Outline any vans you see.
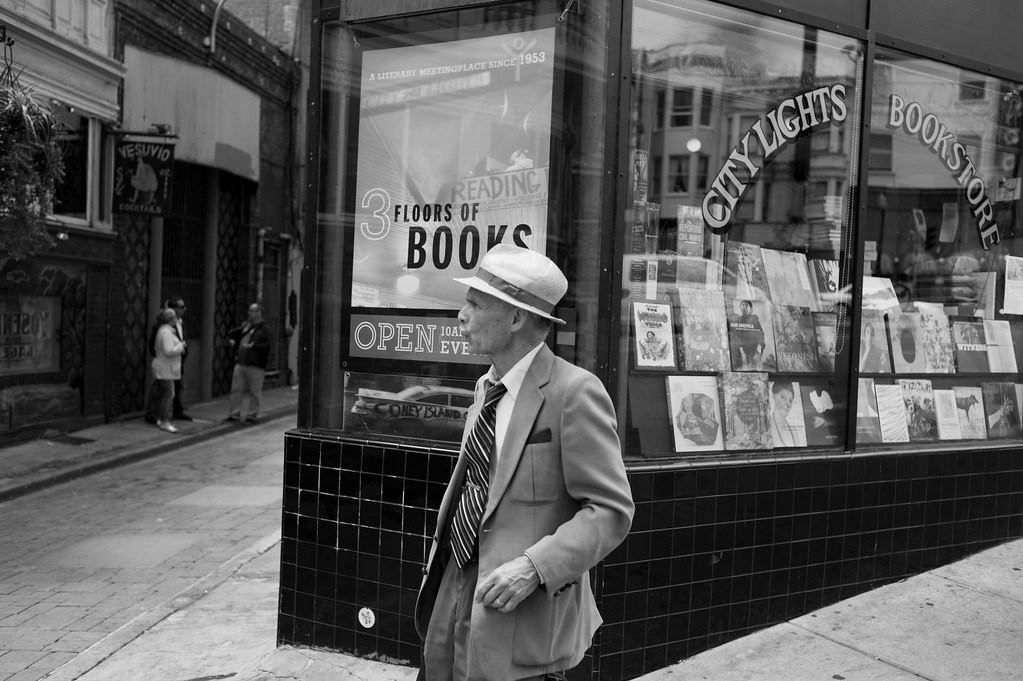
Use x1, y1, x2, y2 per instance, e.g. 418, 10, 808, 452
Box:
622, 253, 774, 335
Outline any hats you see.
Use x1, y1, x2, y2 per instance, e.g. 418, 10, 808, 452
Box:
454, 243, 569, 325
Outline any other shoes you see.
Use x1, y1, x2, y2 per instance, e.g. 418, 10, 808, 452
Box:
149, 408, 254, 432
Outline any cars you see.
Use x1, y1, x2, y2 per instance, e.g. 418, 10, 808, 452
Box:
351, 383, 474, 440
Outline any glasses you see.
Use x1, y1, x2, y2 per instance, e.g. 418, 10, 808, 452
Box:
176, 305, 187, 309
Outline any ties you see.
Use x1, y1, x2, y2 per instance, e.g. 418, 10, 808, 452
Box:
449, 384, 508, 567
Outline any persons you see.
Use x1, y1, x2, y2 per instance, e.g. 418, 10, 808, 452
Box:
858, 322, 885, 373
152, 308, 189, 435
222, 302, 273, 426
413, 243, 637, 681
146, 295, 194, 425
